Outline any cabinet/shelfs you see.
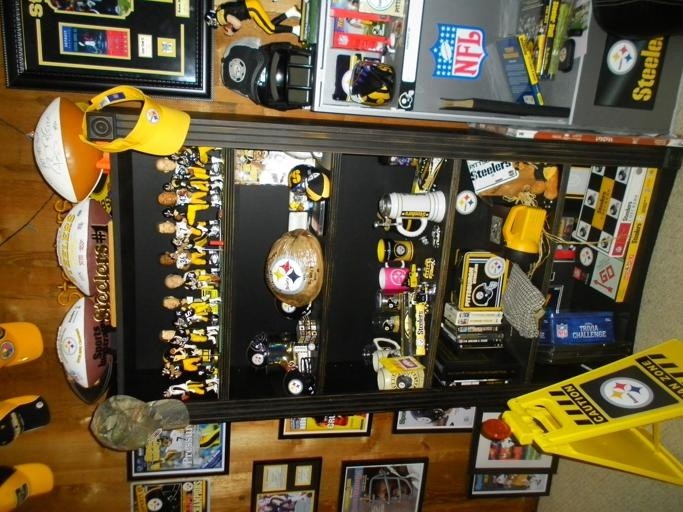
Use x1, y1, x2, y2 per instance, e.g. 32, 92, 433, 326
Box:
90, 113, 683, 422
310, 26, 681, 140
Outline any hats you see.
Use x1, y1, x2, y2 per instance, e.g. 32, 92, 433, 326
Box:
0, 321, 43, 369
78, 84, 191, 156
219, 37, 269, 103
0, 463, 54, 512
88, 395, 191, 453
0, 395, 51, 448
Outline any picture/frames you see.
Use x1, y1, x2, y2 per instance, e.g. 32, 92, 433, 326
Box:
1, 4, 211, 98
127, 407, 559, 511
